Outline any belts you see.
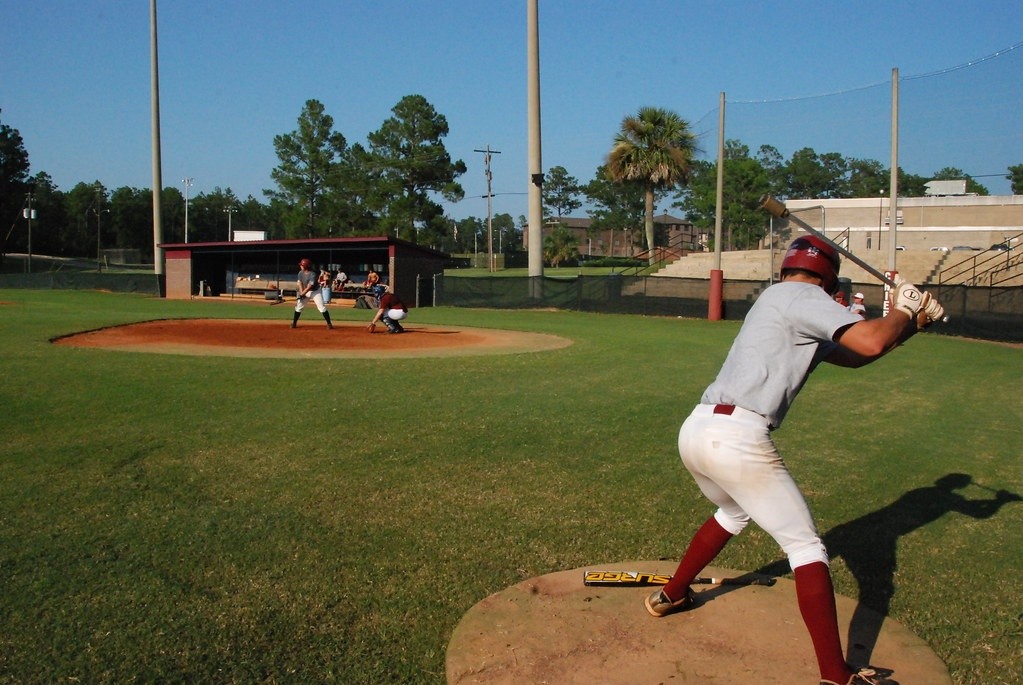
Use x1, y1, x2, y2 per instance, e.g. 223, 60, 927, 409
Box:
714, 404, 735, 415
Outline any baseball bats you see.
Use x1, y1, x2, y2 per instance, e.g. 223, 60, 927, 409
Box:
758, 190, 951, 322
583, 570, 775, 587
269, 298, 300, 306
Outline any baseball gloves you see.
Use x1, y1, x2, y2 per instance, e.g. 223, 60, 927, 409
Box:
367, 323, 376, 333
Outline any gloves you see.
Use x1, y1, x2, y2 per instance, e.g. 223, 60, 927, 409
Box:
917, 290, 943, 328
891, 280, 922, 320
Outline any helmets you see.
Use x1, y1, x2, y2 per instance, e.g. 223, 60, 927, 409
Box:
779, 234, 841, 296
371, 286, 385, 298
299, 259, 310, 268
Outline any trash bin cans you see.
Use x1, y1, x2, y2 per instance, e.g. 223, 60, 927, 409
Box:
200, 280, 207, 296
323, 288, 331, 304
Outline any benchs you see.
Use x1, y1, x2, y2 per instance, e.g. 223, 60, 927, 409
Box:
234, 280, 382, 298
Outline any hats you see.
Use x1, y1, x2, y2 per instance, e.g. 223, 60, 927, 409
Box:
836, 291, 844, 298
854, 293, 864, 299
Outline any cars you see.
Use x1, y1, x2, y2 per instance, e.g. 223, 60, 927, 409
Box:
450, 260, 469, 269
897, 244, 1013, 251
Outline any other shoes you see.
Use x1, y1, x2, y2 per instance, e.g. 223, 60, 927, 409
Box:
328, 323, 334, 329
292, 324, 297, 329
386, 329, 404, 334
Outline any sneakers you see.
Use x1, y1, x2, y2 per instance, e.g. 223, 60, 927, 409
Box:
820, 668, 889, 685
644, 585, 698, 617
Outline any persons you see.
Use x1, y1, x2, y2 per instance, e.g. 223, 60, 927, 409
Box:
367, 285, 409, 333
646, 235, 945, 685
835, 291, 848, 308
363, 269, 379, 289
333, 268, 347, 291
318, 265, 331, 304
290, 259, 333, 330
850, 293, 866, 318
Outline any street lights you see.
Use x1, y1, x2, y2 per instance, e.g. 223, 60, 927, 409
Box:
394, 228, 398, 237
97, 210, 110, 272
222, 209, 238, 242
494, 229, 507, 253
182, 178, 195, 243
878, 190, 884, 250
